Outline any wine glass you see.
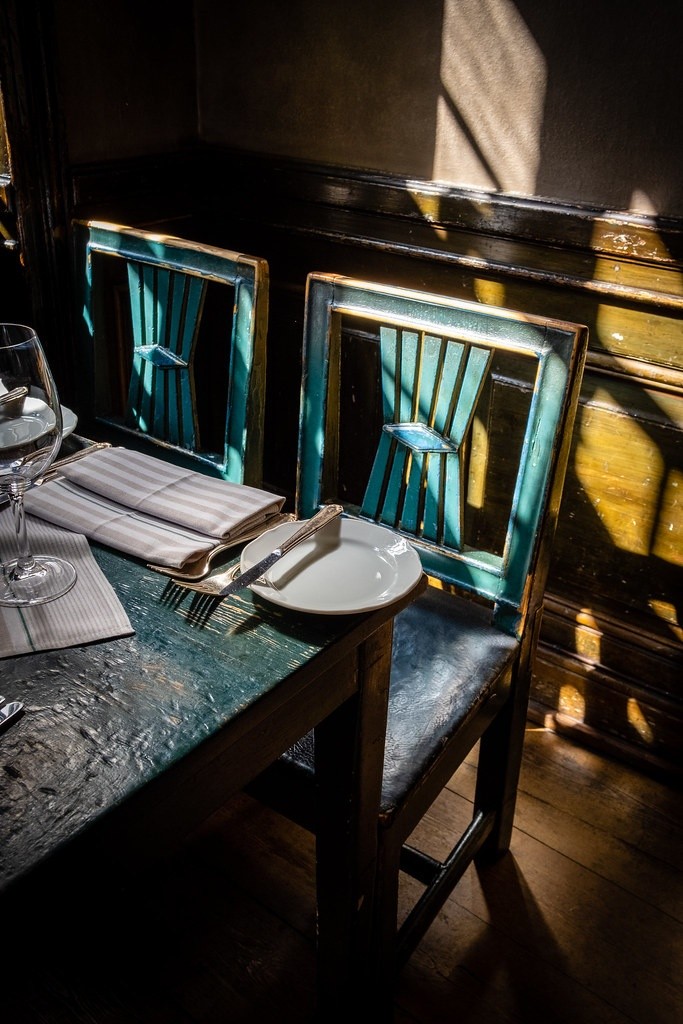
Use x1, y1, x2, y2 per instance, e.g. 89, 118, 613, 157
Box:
0, 324, 77, 609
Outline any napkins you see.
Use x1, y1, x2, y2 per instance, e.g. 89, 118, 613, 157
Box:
1, 445, 294, 659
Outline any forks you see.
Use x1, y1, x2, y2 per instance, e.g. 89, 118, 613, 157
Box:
171, 562, 238, 596
147, 513, 299, 581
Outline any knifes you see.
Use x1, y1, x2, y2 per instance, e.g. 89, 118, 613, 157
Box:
216, 504, 344, 595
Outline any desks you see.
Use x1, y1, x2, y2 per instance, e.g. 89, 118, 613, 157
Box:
0, 435, 431, 1024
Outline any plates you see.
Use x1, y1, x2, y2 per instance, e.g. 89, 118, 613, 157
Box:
0, 396, 78, 449
239, 518, 423, 615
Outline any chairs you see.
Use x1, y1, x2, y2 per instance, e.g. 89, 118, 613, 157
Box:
228, 274, 593, 998
60, 216, 269, 499
